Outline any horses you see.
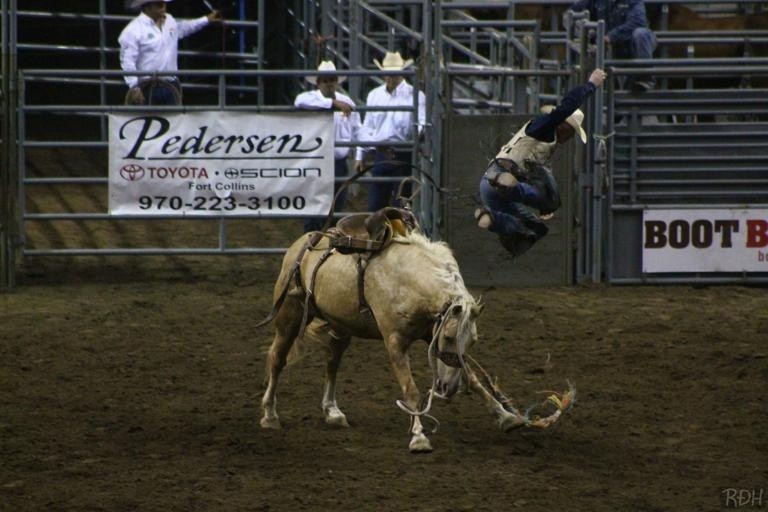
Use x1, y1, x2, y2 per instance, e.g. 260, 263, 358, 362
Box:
258, 228, 527, 453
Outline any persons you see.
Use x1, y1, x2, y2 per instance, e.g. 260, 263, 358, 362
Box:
117, 0, 222, 106
570, 0, 659, 90
361, 51, 426, 220
474, 68, 607, 259
294, 60, 362, 234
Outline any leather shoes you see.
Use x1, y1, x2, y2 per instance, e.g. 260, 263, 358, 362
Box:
474, 208, 494, 229
486, 171, 519, 191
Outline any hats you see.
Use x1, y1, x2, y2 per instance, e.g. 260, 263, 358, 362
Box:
130, 0, 170, 9
373, 52, 414, 70
540, 105, 587, 144
304, 61, 348, 86
